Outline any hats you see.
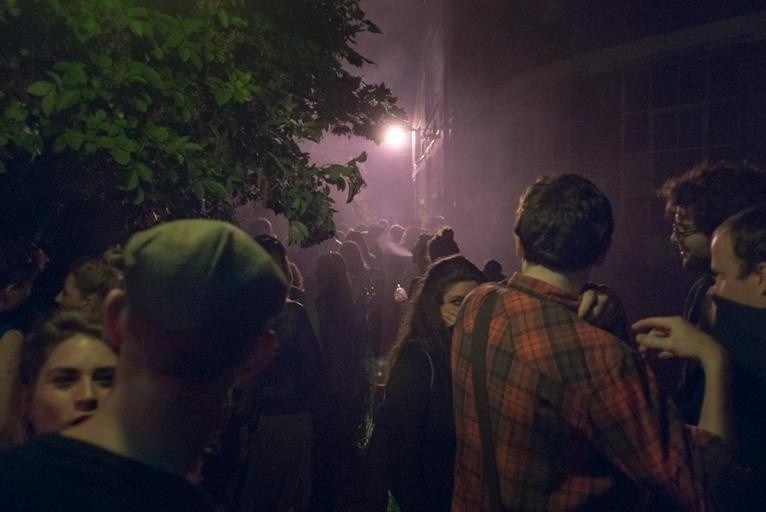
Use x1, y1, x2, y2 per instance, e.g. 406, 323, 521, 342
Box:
125, 218, 288, 340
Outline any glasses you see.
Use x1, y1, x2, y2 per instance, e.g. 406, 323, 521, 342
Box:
673, 223, 701, 235
